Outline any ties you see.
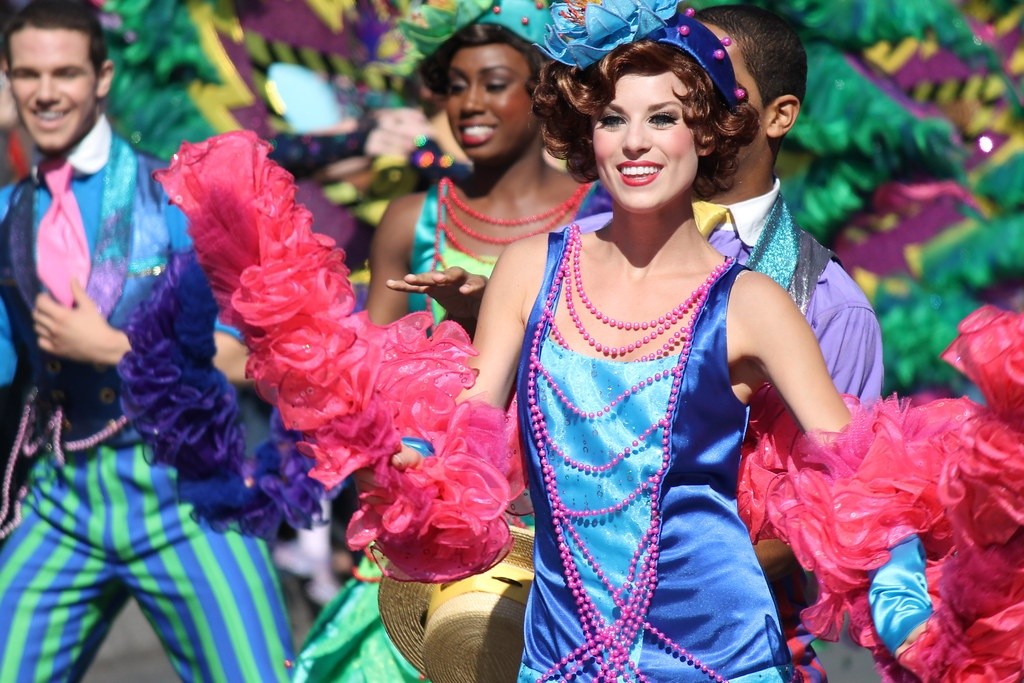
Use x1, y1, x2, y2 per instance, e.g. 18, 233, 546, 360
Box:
37, 160, 90, 310
691, 197, 733, 242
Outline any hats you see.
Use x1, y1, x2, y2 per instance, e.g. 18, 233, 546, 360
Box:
378, 526, 536, 683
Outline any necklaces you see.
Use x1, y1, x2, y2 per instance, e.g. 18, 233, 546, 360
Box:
426, 174, 590, 337
529, 224, 734, 683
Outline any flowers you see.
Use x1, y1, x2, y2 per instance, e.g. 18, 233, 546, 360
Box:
370, 1, 496, 78
530, 0, 677, 69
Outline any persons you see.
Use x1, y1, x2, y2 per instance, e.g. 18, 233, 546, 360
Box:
296, 23, 595, 683
569, 4, 884, 580
0, 0, 298, 683
393, 15, 937, 683
